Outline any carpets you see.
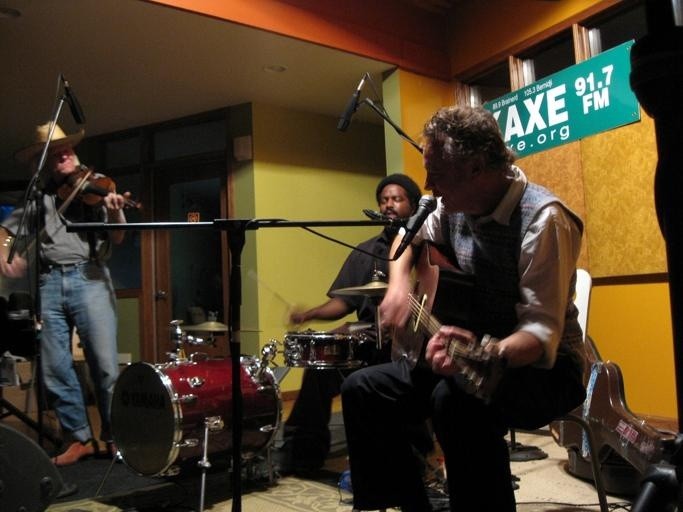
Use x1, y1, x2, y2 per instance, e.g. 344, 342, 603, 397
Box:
45, 412, 633, 511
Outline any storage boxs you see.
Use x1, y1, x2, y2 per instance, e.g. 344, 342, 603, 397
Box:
0, 359, 43, 413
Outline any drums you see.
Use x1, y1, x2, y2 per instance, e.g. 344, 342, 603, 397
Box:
281, 329, 368, 370
109, 352, 281, 480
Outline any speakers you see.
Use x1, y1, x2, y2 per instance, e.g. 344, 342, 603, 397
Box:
0, 423, 64, 512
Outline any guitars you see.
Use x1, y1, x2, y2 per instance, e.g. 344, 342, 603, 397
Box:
391, 243, 511, 405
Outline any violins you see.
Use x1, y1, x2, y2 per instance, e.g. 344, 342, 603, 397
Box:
57, 171, 143, 213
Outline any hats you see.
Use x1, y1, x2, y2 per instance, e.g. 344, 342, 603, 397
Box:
376, 174, 421, 215
18, 120, 84, 158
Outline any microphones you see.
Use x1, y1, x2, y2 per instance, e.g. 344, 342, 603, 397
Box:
393, 194, 437, 260
61, 73, 85, 124
336, 73, 367, 133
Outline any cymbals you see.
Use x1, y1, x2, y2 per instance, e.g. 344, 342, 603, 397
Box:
161, 321, 265, 333
328, 282, 388, 297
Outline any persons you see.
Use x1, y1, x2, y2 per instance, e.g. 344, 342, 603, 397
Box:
628, 0, 683, 430
0, 120, 133, 465
280, 173, 438, 475
341, 103, 586, 512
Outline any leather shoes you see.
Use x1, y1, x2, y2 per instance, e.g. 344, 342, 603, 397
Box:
108, 443, 118, 458
51, 443, 100, 466
